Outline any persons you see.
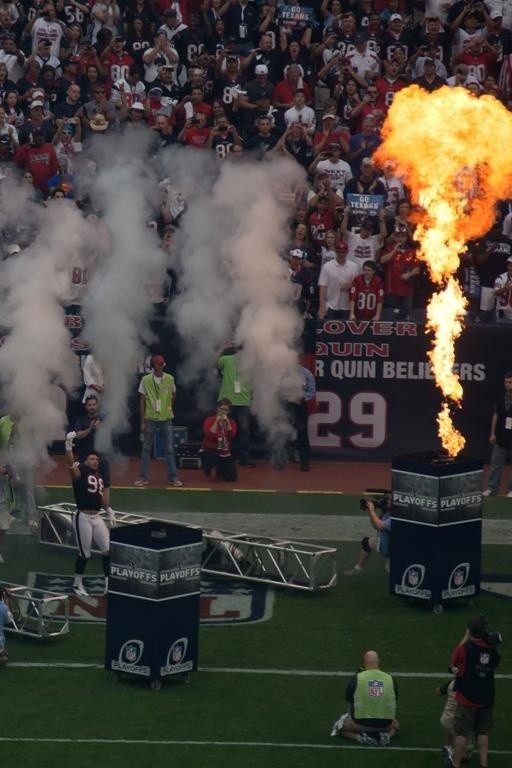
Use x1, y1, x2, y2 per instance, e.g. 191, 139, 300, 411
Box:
316, 240, 358, 319
343, 492, 393, 576
466, 82, 481, 97
272, 65, 316, 109
49, 188, 66, 203
0, 398, 60, 528
474, 224, 512, 324
77, 65, 105, 98
0, 106, 19, 156
292, 202, 307, 223
124, 14, 155, 65
340, 206, 387, 274
61, 21, 87, 58
177, 112, 210, 150
64, 431, 116, 596
380, 0, 405, 19
244, 64, 275, 102
15, 168, 44, 204
123, 0, 154, 33
283, 88, 316, 133
348, 261, 384, 321
3, 91, 23, 126
161, 225, 179, 246
176, 89, 211, 120
321, 230, 339, 263
243, 33, 278, 80
0, 62, 16, 91
350, 117, 382, 160
0, 39, 24, 66
314, 114, 350, 155
247, 117, 279, 152
119, 102, 146, 128
210, 340, 256, 469
28, 39, 61, 79
338, 650, 400, 748
413, 15, 453, 52
111, 65, 145, 112
435, 677, 475, 768
134, 355, 184, 487
199, 398, 238, 482
351, 84, 387, 135
249, 4, 280, 51
51, 124, 83, 175
387, 198, 417, 226
379, 225, 420, 321
52, 55, 82, 117
98, 35, 134, 76
206, 114, 242, 161
25, 93, 50, 125
318, 55, 367, 100
52, 84, 83, 129
72, 395, 111, 509
320, 1, 348, 26
282, 0, 307, 20
204, 1, 226, 33
278, 21, 313, 79
14, 130, 59, 195
492, 255, 512, 324
314, 26, 345, 62
481, 372, 512, 498
151, 113, 176, 147
409, 43, 447, 81
75, 85, 120, 139
317, 98, 347, 138
145, 86, 176, 126
331, 12, 360, 41
391, 46, 408, 59
451, 2, 494, 63
19, 101, 52, 143
47, 158, 79, 203
354, 1, 379, 29
213, 48, 243, 99
413, 59, 448, 90
343, 157, 387, 234
338, 79, 368, 120
42, 66, 57, 92
149, 65, 179, 99
274, 122, 314, 160
308, 143, 355, 200
0, 2, 29, 47
377, 160, 405, 199
451, 617, 501, 768
455, 36, 497, 88
209, 99, 238, 127
180, 0, 211, 69
142, 29, 179, 87
31, 5, 67, 60
157, 8, 190, 63
86, 3, 119, 45
222, 1, 256, 47
373, 59, 406, 113
485, 11, 512, 54
276, 353, 315, 472
15, 60, 41, 94
445, 64, 469, 88
179, 69, 212, 99
484, 75, 496, 89
360, 11, 384, 42
68, 39, 100, 79
282, 249, 314, 319
384, 13, 414, 55
81, 353, 105, 415
281, 223, 314, 267
343, 32, 380, 81
309, 180, 337, 231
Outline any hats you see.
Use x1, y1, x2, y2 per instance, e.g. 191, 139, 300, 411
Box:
489, 9, 502, 19
88, 113, 109, 131
162, 8, 177, 17
335, 242, 347, 252
322, 25, 334, 35
31, 100, 43, 109
427, 40, 439, 48
150, 355, 164, 365
290, 249, 303, 258
383, 160, 395, 167
129, 101, 145, 110
425, 56, 434, 64
322, 112, 335, 120
354, 31, 368, 43
254, 64, 268, 74
390, 13, 401, 22
466, 618, 487, 638
8, 243, 20, 255
394, 225, 407, 236
32, 91, 44, 99
63, 124, 72, 130
361, 219, 374, 231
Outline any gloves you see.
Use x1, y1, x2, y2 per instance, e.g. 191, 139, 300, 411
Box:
65, 430, 76, 451
107, 507, 116, 526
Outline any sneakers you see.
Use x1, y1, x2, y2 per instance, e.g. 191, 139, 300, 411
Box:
442, 745, 454, 768
482, 488, 496, 496
168, 480, 183, 487
343, 566, 364, 577
506, 490, 512, 498
72, 584, 89, 597
357, 731, 378, 747
134, 480, 149, 486
378, 732, 390, 746
28, 520, 37, 527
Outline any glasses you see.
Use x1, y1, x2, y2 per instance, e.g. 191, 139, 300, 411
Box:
95, 90, 105, 94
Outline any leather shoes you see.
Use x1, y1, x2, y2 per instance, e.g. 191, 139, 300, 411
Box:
240, 461, 256, 467
301, 464, 309, 471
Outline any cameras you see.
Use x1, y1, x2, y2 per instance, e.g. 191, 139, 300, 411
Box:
479, 609, 502, 646
360, 489, 392, 512
230, 340, 241, 348
